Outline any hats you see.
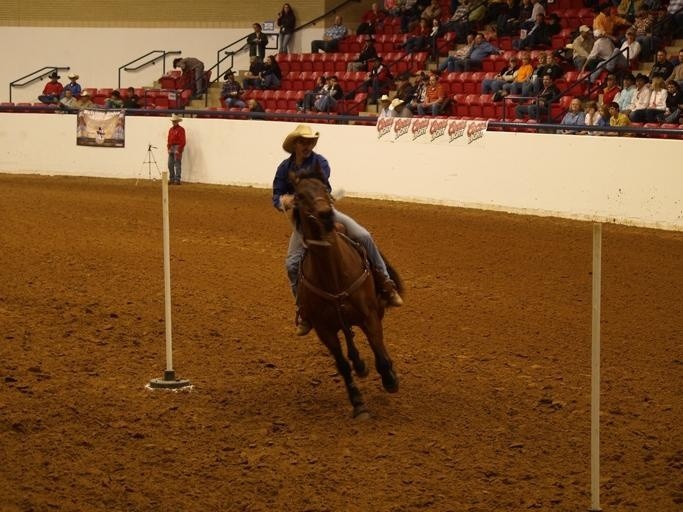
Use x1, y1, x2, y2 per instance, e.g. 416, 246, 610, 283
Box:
67, 73, 79, 79
172, 57, 182, 68
579, 24, 590, 33
224, 70, 237, 80
281, 124, 320, 152
169, 113, 183, 122
48, 73, 60, 79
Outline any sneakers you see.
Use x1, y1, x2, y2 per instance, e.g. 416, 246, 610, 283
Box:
167, 180, 181, 185
381, 281, 404, 307
295, 320, 312, 337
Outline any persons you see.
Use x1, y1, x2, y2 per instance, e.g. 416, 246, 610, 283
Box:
123, 87, 140, 114
356, 1, 440, 52
296, 75, 343, 112
174, 57, 204, 100
38, 72, 94, 114
272, 125, 404, 336
278, 3, 295, 54
377, 70, 446, 117
104, 91, 123, 112
167, 115, 185, 185
221, 23, 281, 117
556, 1, 683, 135
368, 58, 393, 97
347, 41, 376, 73
430, 1, 562, 121
311, 15, 346, 53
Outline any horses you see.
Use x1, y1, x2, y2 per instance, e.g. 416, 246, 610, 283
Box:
287, 155, 404, 423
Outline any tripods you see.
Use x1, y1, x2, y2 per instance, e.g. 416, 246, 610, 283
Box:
135, 144, 162, 185
